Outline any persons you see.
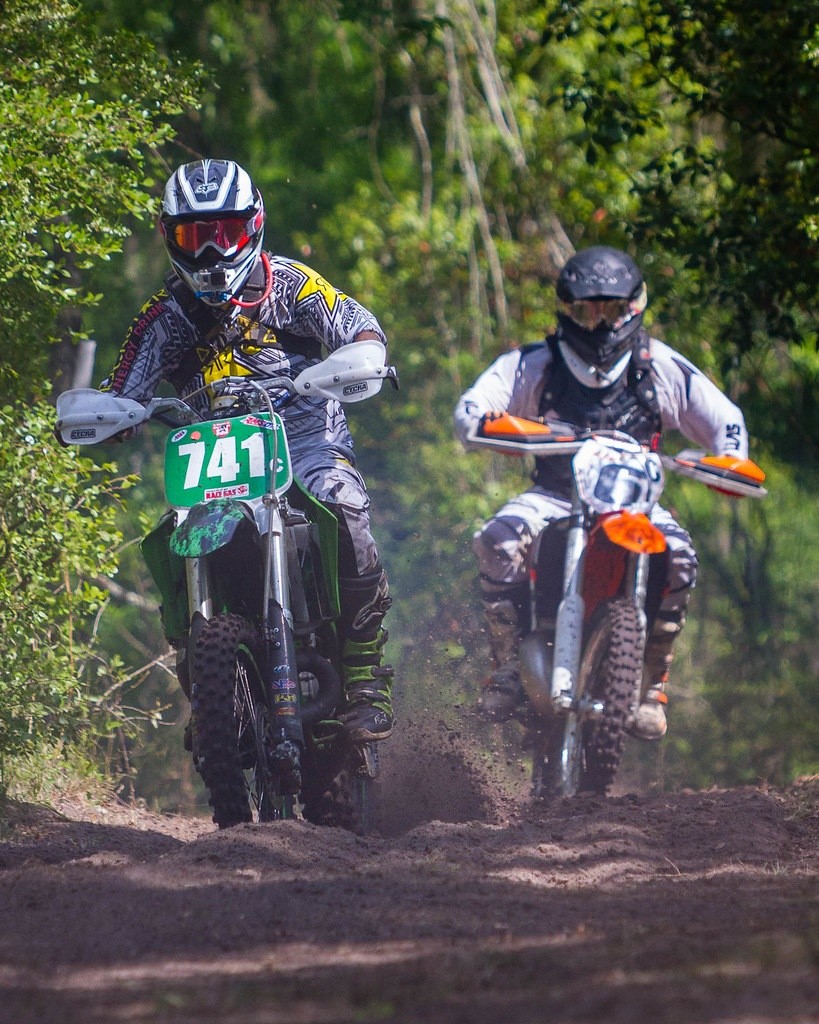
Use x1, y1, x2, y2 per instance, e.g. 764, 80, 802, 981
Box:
455, 244, 750, 741
54, 155, 396, 748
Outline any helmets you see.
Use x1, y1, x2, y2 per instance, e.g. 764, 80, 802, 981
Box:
554, 246, 648, 406
158, 158, 264, 307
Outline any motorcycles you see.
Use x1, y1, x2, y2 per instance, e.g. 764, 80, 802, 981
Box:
466, 411, 769, 800
53, 341, 403, 827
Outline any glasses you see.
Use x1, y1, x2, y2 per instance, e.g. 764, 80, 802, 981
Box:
568, 298, 631, 331
161, 207, 253, 259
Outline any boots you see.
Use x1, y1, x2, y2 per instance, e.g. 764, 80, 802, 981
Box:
176, 639, 193, 751
479, 574, 533, 718
336, 567, 395, 743
632, 610, 687, 743
